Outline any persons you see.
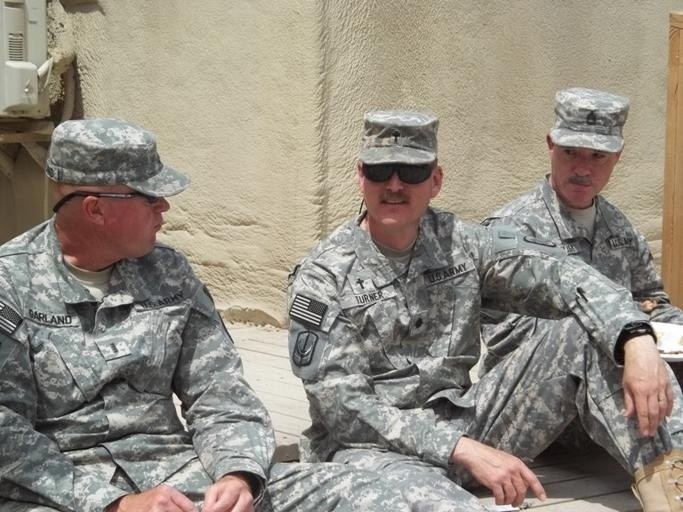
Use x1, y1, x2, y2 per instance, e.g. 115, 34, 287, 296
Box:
284, 110, 681, 511
474, 86, 683, 474
0, 116, 411, 511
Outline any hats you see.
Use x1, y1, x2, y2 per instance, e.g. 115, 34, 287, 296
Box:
44, 117, 191, 198
549, 86, 630, 153
360, 110, 439, 166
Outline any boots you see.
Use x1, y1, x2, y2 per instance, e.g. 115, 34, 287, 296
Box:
630, 448, 683, 512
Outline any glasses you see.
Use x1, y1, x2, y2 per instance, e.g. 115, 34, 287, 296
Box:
52, 190, 159, 213
362, 163, 432, 184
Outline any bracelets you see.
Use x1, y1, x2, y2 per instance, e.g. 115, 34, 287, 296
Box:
615, 321, 657, 365
